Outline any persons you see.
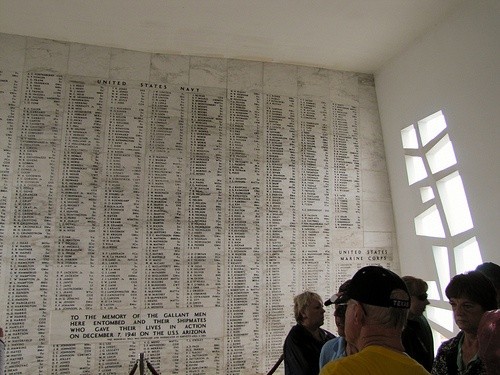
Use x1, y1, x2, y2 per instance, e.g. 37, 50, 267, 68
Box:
282, 290, 337, 375
433, 270, 500, 375
318, 265, 435, 375
474, 261, 500, 310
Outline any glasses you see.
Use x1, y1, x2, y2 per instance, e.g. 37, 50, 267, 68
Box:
414, 294, 427, 301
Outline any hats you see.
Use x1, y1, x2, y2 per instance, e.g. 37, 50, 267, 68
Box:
324, 266, 411, 309
